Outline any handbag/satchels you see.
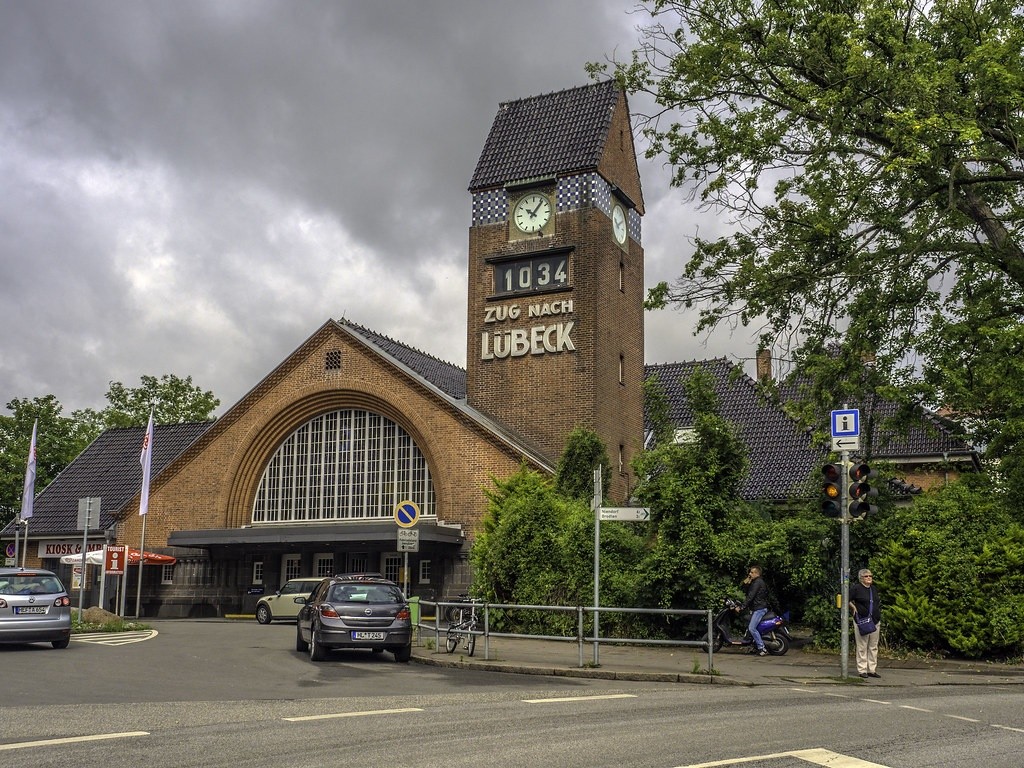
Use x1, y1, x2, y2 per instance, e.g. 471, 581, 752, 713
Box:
857, 616, 877, 636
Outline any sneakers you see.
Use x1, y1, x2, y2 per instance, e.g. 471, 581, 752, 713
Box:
754, 648, 769, 656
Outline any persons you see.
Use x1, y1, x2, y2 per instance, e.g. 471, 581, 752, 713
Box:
736, 565, 772, 657
848, 569, 883, 679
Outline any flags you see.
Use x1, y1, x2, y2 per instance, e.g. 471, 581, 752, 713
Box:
20, 417, 37, 523
138, 407, 153, 516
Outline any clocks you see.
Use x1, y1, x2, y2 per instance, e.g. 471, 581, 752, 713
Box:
609, 193, 631, 255
508, 184, 556, 242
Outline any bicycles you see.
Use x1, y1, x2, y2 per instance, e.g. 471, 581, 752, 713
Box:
446, 598, 482, 656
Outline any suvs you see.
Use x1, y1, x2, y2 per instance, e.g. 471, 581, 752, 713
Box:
0, 567, 72, 649
294, 574, 412, 664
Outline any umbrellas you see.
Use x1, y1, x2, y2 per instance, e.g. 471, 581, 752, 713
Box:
60, 545, 177, 616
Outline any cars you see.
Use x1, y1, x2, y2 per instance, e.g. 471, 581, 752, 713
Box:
254, 577, 330, 624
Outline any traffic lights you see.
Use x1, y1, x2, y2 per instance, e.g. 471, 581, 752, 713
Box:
845, 464, 879, 521
821, 464, 842, 518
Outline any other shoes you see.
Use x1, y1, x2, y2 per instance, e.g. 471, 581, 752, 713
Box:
860, 673, 881, 678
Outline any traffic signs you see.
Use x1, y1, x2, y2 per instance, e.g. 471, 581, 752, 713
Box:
831, 409, 860, 452
5, 558, 15, 566
599, 506, 651, 522
397, 527, 419, 552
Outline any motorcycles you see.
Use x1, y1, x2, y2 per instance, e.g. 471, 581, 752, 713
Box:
702, 599, 792, 656
